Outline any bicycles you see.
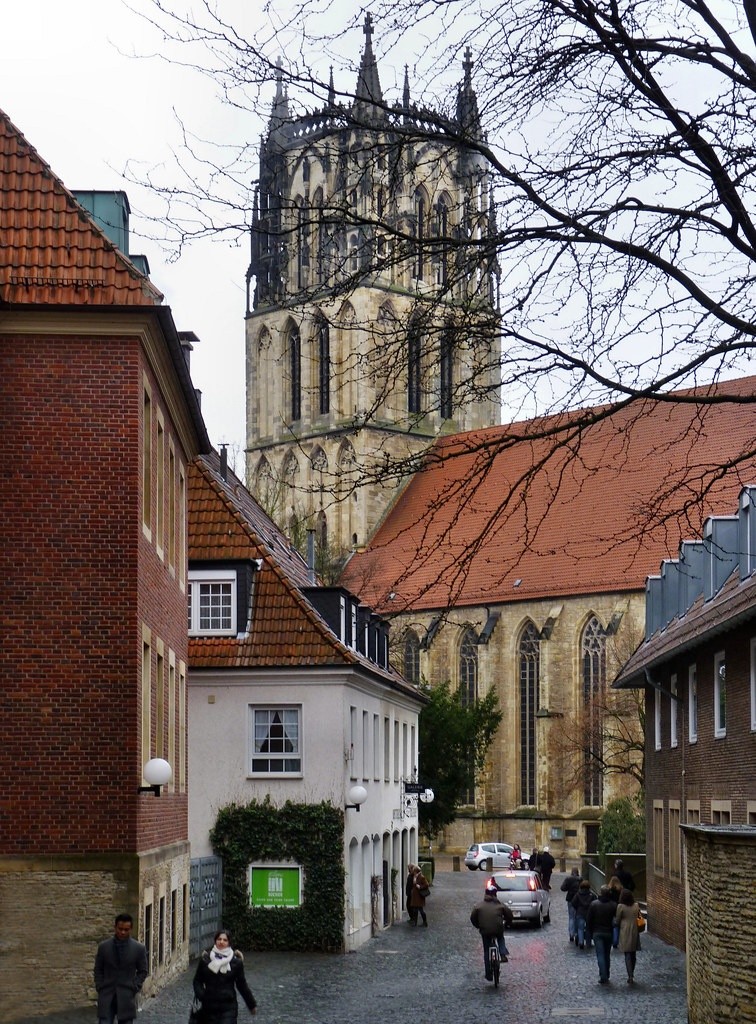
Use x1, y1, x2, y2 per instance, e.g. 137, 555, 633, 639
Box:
487, 936, 504, 989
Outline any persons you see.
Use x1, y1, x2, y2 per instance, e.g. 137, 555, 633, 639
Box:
193, 930, 258, 1024
508, 844, 555, 892
470, 885, 513, 981
406, 863, 430, 927
94, 913, 149, 1024
560, 865, 644, 986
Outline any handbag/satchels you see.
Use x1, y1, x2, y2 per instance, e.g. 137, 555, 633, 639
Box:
419, 887, 430, 897
533, 866, 541, 873
544, 847, 549, 852
188, 996, 201, 1024
637, 911, 645, 932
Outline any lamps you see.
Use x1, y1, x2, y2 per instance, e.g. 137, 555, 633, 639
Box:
344, 786, 367, 812
138, 759, 172, 797
412, 790, 434, 807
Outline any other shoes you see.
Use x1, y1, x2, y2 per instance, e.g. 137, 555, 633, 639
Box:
407, 919, 428, 927
485, 975, 493, 981
598, 979, 609, 984
502, 957, 508, 962
570, 935, 585, 950
627, 979, 633, 983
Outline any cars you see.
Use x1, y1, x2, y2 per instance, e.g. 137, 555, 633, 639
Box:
484, 870, 552, 929
465, 842, 532, 872
